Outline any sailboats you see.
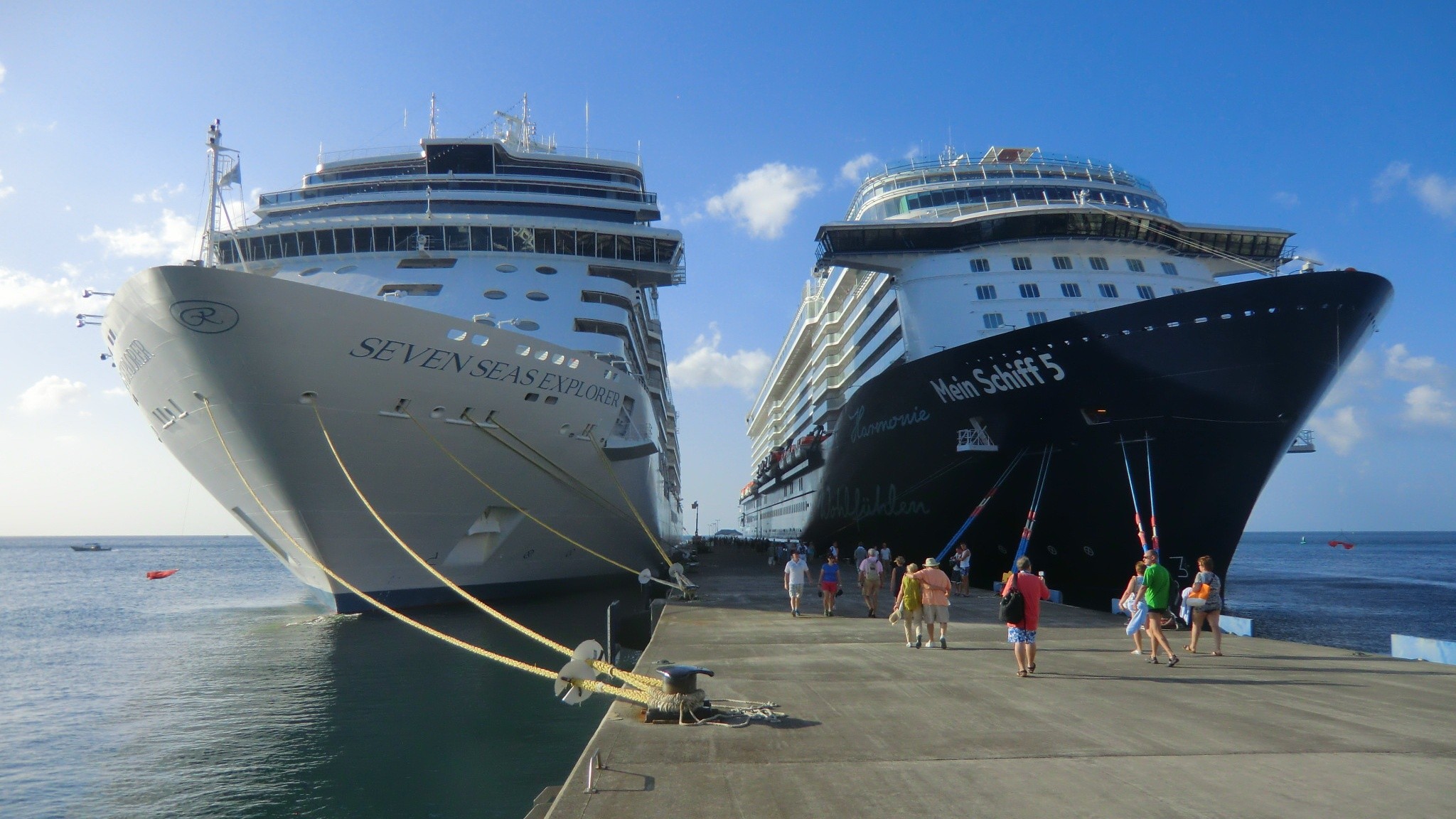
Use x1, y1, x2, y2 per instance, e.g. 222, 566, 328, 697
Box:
1300, 535, 1306, 544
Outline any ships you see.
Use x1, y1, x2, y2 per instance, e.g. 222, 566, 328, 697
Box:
78, 93, 691, 615
739, 142, 1393, 627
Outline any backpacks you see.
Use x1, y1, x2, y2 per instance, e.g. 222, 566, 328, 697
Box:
866, 558, 879, 581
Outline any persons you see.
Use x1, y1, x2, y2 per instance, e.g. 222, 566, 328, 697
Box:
1183, 555, 1223, 657
702, 536, 971, 649
1119, 550, 1180, 667
999, 557, 1050, 677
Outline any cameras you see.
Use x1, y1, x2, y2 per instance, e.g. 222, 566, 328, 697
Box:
1039, 571, 1044, 576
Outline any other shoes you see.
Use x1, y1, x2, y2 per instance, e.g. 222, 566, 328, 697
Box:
1144, 656, 1158, 664
824, 610, 833, 617
1130, 649, 1144, 654
791, 608, 800, 616
916, 633, 922, 648
1017, 670, 1027, 677
940, 636, 946, 649
868, 608, 876, 618
906, 642, 912, 647
1027, 662, 1035, 673
1212, 650, 1224, 656
1165, 654, 1179, 667
925, 642, 935, 647
1183, 645, 1197, 654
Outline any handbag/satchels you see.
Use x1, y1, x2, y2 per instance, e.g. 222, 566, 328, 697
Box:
899, 599, 904, 619
1186, 573, 1215, 606
999, 573, 1026, 634
1123, 593, 1137, 608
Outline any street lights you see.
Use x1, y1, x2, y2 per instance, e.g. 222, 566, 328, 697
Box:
708, 520, 720, 540
691, 503, 698, 544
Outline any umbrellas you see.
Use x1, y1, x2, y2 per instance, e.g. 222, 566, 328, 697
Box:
715, 529, 742, 535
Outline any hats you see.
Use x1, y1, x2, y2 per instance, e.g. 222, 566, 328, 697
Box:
894, 556, 906, 564
889, 608, 901, 626
836, 589, 843, 598
922, 558, 941, 569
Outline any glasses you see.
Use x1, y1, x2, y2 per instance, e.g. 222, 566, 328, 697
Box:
1143, 556, 1152, 559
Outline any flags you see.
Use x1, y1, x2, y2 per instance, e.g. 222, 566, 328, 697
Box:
220, 164, 241, 186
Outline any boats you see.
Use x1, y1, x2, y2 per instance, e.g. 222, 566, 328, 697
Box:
740, 479, 759, 499
757, 436, 826, 483
72, 543, 111, 552
